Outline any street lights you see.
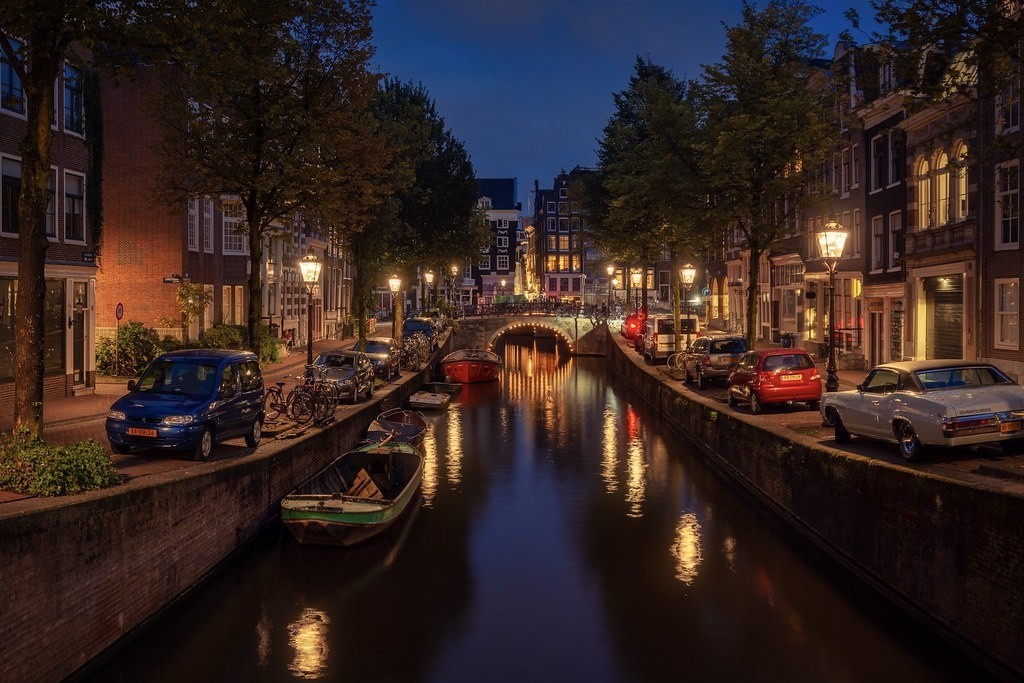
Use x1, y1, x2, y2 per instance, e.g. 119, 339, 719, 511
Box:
605, 209, 849, 393
298, 248, 459, 365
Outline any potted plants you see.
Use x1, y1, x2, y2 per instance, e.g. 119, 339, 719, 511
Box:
778, 331, 794, 348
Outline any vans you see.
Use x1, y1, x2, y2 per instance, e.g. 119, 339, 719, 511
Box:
634, 313, 701, 364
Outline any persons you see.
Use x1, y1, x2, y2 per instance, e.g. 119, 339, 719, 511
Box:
286, 329, 292, 348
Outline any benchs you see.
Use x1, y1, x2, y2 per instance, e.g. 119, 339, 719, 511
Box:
924, 381, 967, 389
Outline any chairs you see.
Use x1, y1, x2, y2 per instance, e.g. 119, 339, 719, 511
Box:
784, 356, 794, 367
204, 374, 223, 390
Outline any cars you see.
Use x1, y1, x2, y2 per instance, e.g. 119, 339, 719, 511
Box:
105, 348, 266, 462
819, 360, 1024, 460
621, 313, 648, 339
682, 333, 751, 389
302, 351, 375, 404
726, 348, 823, 415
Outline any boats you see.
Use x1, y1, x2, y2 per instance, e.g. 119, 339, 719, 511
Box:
409, 382, 462, 409
281, 441, 425, 544
364, 408, 428, 449
439, 349, 502, 383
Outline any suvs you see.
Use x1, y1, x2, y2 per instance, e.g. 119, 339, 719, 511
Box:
402, 317, 439, 352
349, 337, 400, 380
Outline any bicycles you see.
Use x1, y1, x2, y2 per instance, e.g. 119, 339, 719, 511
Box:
399, 331, 429, 371
106, 357, 138, 379
667, 347, 688, 371
264, 375, 315, 424
286, 367, 340, 421
